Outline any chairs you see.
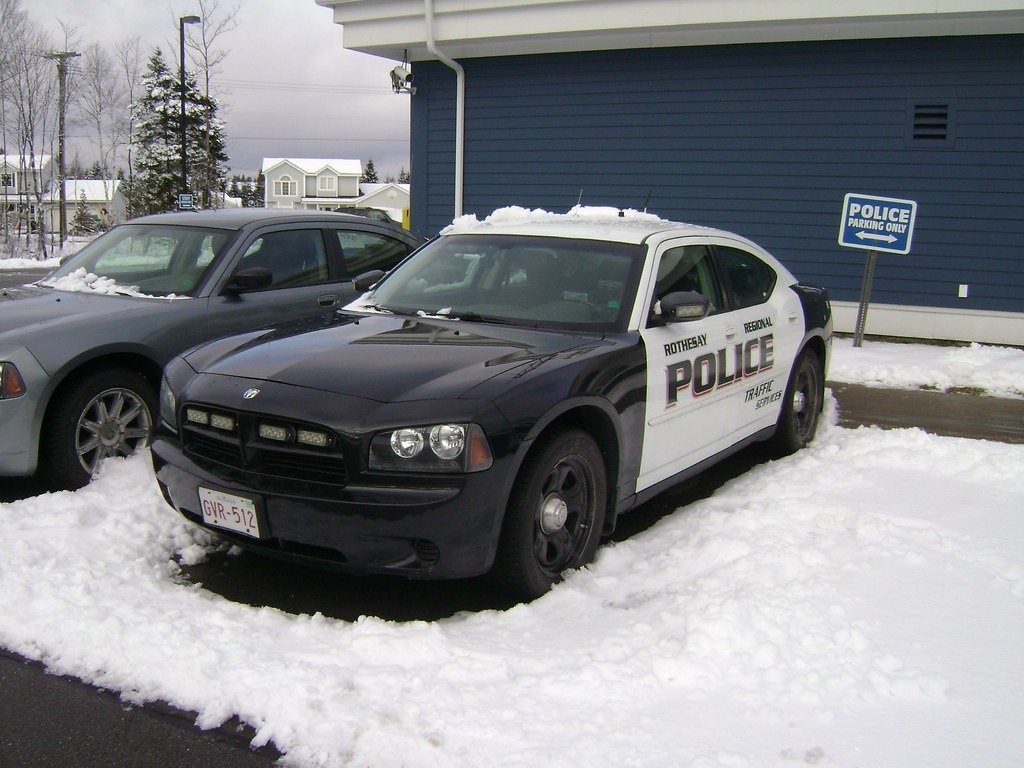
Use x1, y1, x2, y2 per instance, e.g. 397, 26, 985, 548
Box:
500, 250, 561, 303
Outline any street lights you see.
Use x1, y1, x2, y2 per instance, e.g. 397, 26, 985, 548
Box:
181, 16, 202, 194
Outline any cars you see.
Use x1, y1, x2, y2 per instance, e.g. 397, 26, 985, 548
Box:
148, 216, 834, 605
334, 208, 402, 225
1, 208, 471, 491
